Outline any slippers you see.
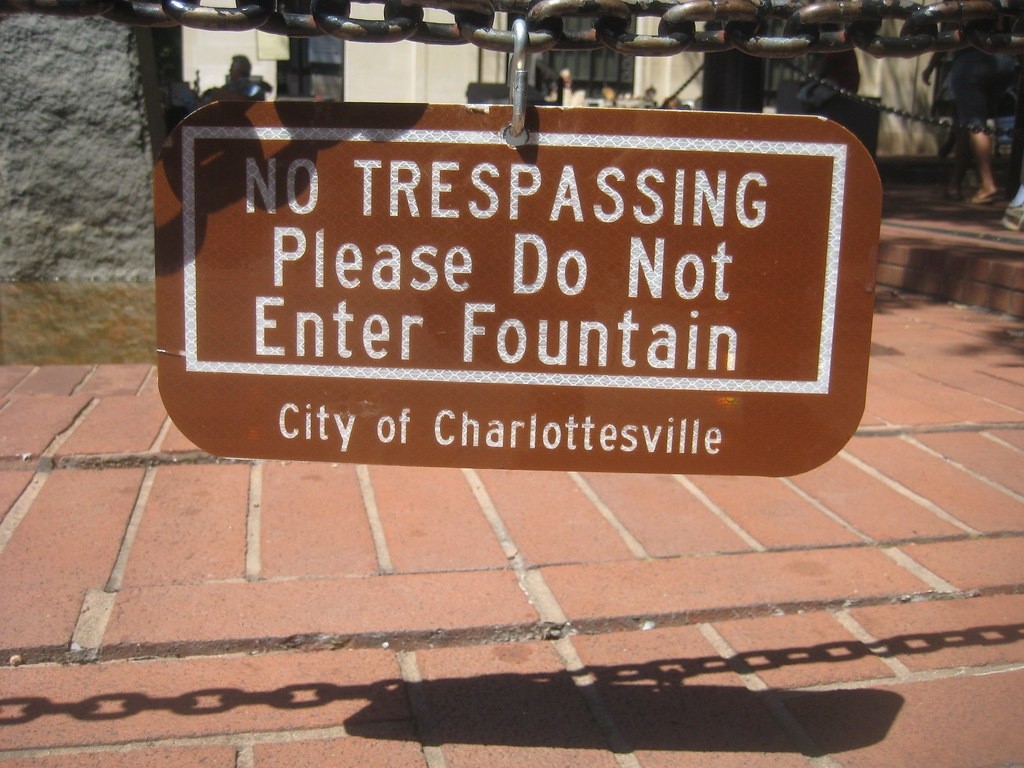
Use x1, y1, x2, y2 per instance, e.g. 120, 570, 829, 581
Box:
966, 192, 996, 204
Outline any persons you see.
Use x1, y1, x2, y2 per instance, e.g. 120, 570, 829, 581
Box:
200, 55, 252, 108
921, 0, 1024, 206
